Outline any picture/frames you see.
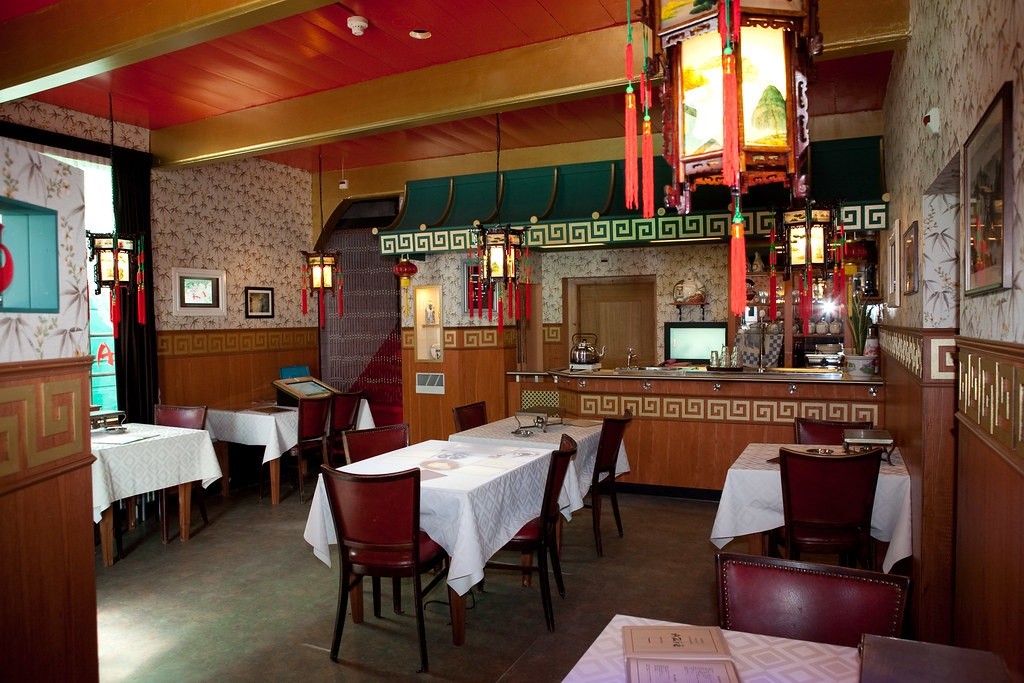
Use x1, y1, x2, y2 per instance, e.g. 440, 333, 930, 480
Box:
245, 286, 274, 319
887, 219, 900, 307
963, 80, 1013, 296
903, 220, 919, 296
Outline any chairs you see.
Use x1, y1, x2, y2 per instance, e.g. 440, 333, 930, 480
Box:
452, 401, 488, 433
257, 397, 332, 505
794, 416, 873, 446
714, 552, 910, 649
341, 424, 443, 614
301, 390, 364, 480
477, 434, 578, 631
767, 447, 882, 570
138, 404, 208, 545
321, 464, 452, 673
555, 408, 634, 557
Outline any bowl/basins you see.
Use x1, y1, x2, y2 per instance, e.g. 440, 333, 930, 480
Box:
805, 343, 844, 362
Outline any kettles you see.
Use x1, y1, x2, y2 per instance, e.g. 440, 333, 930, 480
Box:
570, 333, 606, 364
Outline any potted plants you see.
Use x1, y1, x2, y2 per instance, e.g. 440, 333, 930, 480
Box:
844, 291, 879, 380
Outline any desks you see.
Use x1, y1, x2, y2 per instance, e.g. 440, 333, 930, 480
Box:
561, 614, 862, 683
303, 440, 572, 647
448, 416, 631, 552
91, 423, 222, 567
203, 398, 376, 506
709, 442, 912, 575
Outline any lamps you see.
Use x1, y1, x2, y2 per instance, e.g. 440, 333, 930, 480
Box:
624, 0, 824, 317
469, 115, 530, 335
86, 94, 146, 339
769, 145, 844, 336
300, 145, 343, 329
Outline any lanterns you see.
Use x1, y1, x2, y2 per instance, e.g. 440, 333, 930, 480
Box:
624, 0, 823, 318
843, 242, 867, 318
86, 230, 147, 338
301, 250, 346, 329
392, 254, 418, 317
769, 197, 847, 338
468, 221, 532, 333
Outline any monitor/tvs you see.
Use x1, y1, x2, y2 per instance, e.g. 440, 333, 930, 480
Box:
664, 322, 728, 366
285, 380, 332, 396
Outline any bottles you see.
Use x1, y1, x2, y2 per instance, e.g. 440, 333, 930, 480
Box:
628, 349, 638, 369
863, 326, 878, 373
752, 252, 764, 272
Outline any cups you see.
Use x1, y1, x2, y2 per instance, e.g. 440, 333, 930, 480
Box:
710, 345, 743, 367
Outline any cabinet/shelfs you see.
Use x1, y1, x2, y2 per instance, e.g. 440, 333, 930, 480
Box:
727, 231, 880, 369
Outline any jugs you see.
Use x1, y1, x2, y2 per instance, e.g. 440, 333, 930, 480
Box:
808, 319, 843, 333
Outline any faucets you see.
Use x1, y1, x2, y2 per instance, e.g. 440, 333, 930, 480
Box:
628, 346, 636, 367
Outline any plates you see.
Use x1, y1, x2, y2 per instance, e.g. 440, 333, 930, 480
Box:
806, 449, 834, 454
511, 430, 533, 437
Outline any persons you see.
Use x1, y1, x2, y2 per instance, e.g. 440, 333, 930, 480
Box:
675, 271, 706, 302
745, 278, 761, 304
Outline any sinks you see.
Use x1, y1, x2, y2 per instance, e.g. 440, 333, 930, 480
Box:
613, 364, 697, 373
